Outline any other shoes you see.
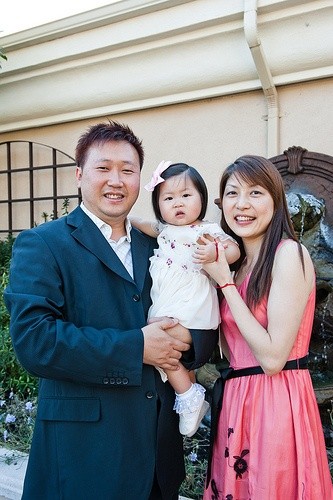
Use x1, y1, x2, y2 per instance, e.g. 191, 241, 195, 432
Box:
173, 383, 206, 435
187, 399, 210, 437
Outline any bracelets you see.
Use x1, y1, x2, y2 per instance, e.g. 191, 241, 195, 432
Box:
217, 283, 235, 289
215, 244, 218, 261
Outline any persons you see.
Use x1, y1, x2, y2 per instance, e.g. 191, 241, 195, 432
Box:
129, 163, 240, 437
3, 121, 190, 500
203, 155, 333, 500
